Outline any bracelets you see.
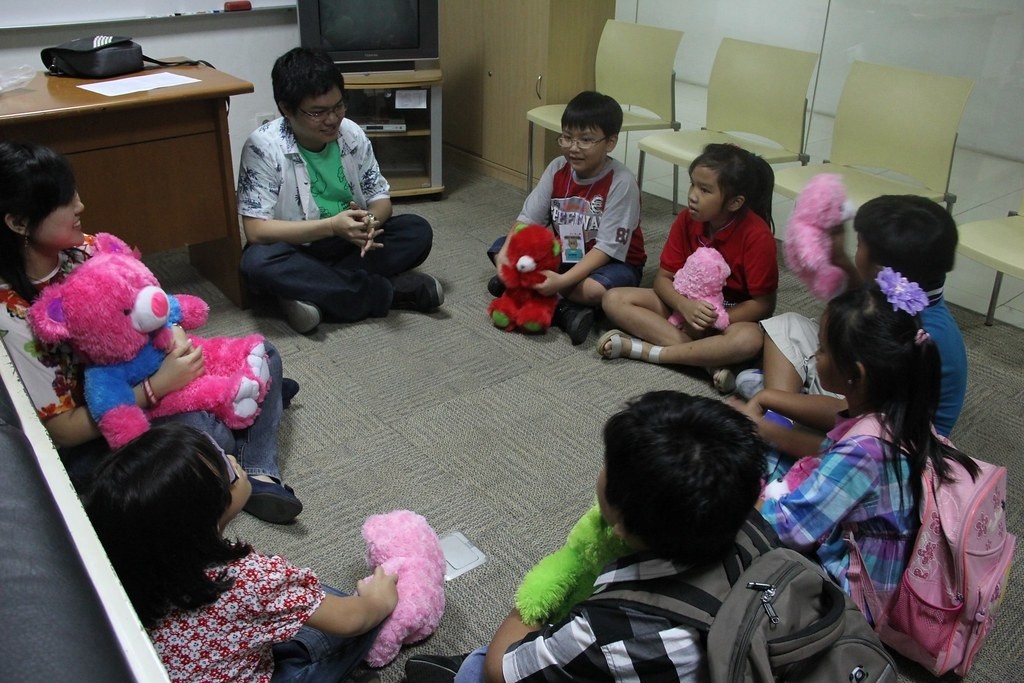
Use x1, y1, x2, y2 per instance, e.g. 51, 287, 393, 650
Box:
367, 214, 377, 223
142, 376, 158, 407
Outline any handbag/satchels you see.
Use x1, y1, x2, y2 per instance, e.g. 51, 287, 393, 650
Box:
40, 34, 145, 79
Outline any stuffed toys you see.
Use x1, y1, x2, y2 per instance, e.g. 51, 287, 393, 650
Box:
758, 455, 822, 513
781, 173, 858, 302
487, 223, 562, 337
352, 510, 445, 670
667, 247, 732, 332
514, 496, 632, 629
30, 233, 273, 452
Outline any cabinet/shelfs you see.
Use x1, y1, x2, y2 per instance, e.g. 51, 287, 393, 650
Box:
0, 55, 247, 305
338, 69, 445, 201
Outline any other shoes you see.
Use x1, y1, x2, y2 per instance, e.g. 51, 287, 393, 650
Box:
406, 654, 460, 683
557, 306, 595, 346
487, 275, 507, 298
278, 293, 322, 333
239, 475, 303, 525
735, 369, 810, 404
280, 377, 300, 399
383, 270, 445, 312
342, 660, 381, 683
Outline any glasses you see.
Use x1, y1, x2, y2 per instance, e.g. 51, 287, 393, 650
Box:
299, 97, 349, 122
201, 429, 241, 484
555, 130, 609, 149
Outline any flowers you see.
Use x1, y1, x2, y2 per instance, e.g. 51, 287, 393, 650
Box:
876, 263, 927, 314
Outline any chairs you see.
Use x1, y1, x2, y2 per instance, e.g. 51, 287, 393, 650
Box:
772, 60, 975, 219
637, 37, 819, 197
525, 18, 684, 216
956, 202, 1024, 325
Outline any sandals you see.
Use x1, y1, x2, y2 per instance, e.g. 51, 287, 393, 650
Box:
705, 362, 751, 394
596, 330, 665, 366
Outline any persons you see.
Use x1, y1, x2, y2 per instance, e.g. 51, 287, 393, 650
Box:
403, 390, 784, 683
238, 46, 444, 333
751, 264, 981, 631
731, 194, 968, 440
488, 91, 647, 346
0, 139, 303, 525
76, 423, 400, 683
595, 143, 777, 395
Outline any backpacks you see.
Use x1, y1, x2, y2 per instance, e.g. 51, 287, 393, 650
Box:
587, 509, 902, 683
834, 412, 1014, 674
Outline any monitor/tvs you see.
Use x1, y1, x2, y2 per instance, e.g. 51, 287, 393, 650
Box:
298, 0, 439, 76
